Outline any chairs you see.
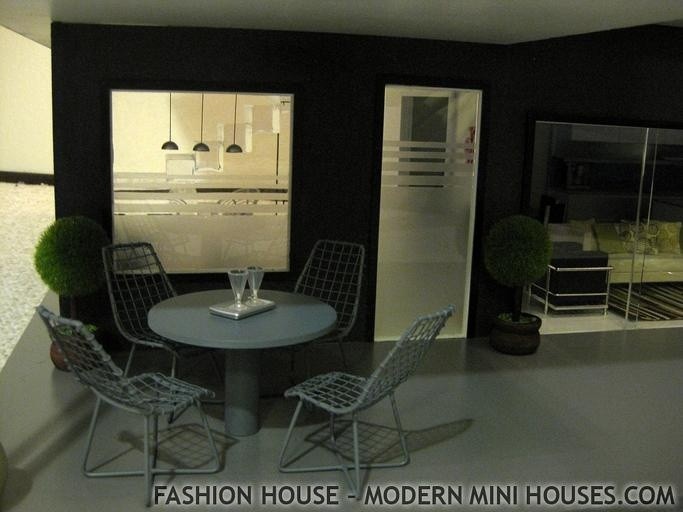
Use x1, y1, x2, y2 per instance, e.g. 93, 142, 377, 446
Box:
254, 236, 457, 500
32, 238, 225, 508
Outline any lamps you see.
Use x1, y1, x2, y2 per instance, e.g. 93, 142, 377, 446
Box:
160, 90, 244, 154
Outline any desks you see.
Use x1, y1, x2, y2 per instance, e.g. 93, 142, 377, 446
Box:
146, 287, 338, 439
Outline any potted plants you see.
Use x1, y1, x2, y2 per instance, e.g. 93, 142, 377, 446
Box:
31, 214, 115, 371
480, 214, 552, 356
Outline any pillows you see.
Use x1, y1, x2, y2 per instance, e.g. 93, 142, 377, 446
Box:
562, 217, 681, 259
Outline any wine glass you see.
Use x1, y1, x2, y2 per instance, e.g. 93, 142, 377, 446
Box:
247, 265, 266, 308
227, 266, 250, 312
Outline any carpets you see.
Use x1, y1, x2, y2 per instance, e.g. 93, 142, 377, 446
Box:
606, 282, 682, 323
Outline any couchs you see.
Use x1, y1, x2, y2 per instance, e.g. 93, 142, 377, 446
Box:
544, 216, 682, 286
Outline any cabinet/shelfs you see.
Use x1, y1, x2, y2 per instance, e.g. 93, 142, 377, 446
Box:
525, 261, 615, 317
